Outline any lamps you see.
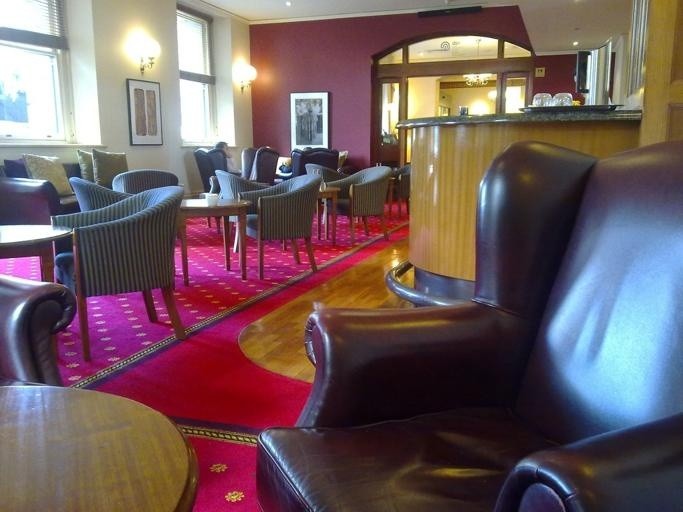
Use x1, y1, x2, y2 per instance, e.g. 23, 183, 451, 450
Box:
464, 38, 493, 86
138, 37, 161, 75
239, 64, 256, 90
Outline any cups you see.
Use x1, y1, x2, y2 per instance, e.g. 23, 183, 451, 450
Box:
205, 193, 219, 206
532, 92, 552, 107
459, 106, 468, 116
552, 92, 574, 107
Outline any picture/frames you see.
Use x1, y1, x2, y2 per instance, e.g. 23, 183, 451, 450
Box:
288, 91, 329, 153
127, 79, 163, 146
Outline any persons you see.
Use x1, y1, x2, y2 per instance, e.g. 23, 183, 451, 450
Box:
295, 98, 322, 145
215, 142, 243, 174
